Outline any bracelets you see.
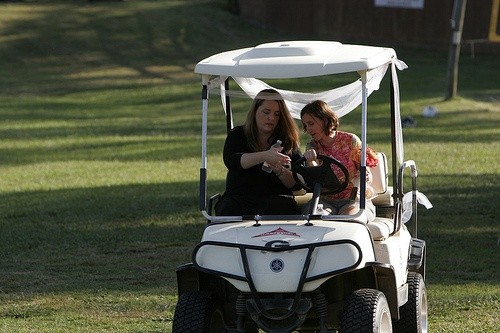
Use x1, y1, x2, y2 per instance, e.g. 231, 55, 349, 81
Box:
277, 166, 285, 178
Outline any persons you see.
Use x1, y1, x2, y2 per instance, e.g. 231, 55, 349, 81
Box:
300, 100, 379, 224
214, 89, 307, 217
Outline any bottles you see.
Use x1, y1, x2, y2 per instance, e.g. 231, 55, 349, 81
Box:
262, 140, 282, 173
